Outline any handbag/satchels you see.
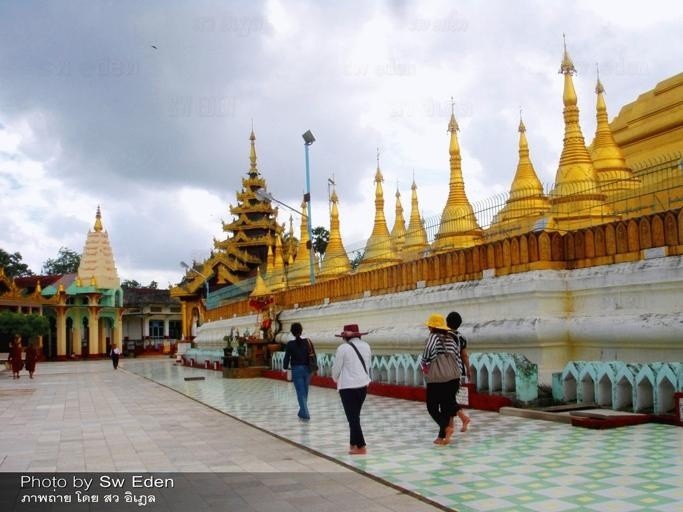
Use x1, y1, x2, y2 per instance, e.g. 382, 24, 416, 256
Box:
455, 387, 469, 406
308, 351, 317, 371
423, 352, 461, 383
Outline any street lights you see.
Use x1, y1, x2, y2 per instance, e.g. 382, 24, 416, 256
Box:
255, 129, 315, 291
179, 261, 209, 298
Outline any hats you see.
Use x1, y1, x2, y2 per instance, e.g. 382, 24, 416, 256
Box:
424, 313, 452, 331
335, 325, 368, 337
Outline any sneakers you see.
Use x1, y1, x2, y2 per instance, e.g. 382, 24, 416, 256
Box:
433, 412, 470, 446
348, 447, 368, 455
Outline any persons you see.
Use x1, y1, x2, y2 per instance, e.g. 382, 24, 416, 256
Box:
420, 314, 463, 446
444, 312, 474, 439
329, 322, 374, 456
8, 334, 23, 380
24, 338, 39, 380
109, 344, 120, 370
282, 320, 320, 422
